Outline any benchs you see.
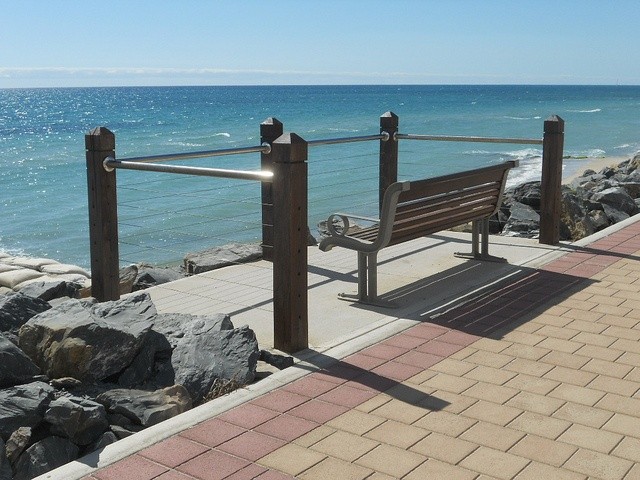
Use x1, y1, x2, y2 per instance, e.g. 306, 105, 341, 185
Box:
318, 156, 521, 311
